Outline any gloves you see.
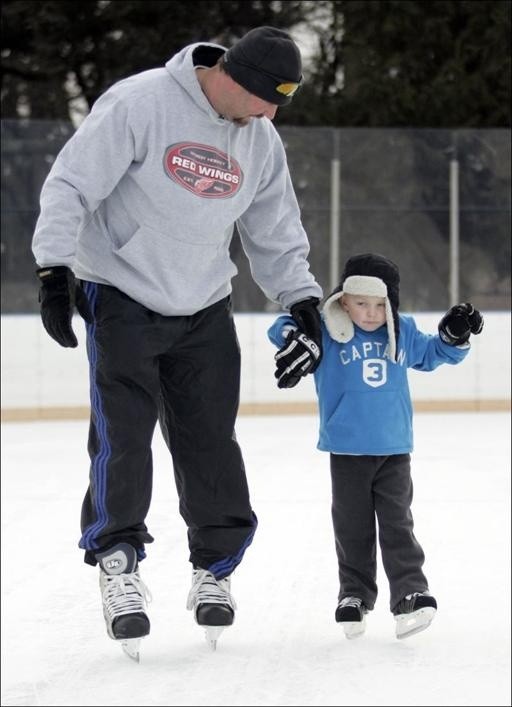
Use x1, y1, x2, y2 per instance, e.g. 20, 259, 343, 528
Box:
36, 266, 93, 348
274, 297, 325, 389
437, 302, 489, 347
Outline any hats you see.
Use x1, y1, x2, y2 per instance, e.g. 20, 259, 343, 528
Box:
221, 25, 302, 110
323, 252, 400, 362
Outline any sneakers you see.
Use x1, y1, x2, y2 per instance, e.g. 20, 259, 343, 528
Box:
94, 541, 150, 640
336, 595, 364, 622
388, 592, 437, 614
185, 568, 234, 626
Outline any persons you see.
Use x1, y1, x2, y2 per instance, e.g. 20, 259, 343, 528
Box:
264, 249, 487, 624
28, 22, 325, 647
